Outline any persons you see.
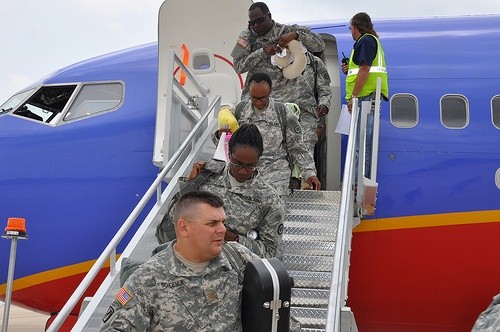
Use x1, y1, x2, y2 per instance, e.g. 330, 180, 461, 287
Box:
335, 13, 389, 191
209, 2, 332, 215
98, 192, 301, 332
169, 124, 284, 261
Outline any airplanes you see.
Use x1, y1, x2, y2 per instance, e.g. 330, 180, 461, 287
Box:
0, 0, 500, 332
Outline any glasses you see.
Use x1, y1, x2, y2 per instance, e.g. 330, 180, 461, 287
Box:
249, 91, 269, 102
248, 12, 269, 26
230, 153, 258, 172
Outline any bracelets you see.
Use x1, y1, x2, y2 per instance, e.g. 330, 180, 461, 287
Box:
350, 95, 357, 99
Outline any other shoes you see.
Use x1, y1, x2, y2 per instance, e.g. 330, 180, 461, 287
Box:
300, 177, 311, 190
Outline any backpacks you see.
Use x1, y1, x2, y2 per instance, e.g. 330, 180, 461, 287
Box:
154, 159, 226, 244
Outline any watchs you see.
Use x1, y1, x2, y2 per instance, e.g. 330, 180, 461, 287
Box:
295, 31, 300, 40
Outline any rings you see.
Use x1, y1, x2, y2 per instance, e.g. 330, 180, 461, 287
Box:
323, 112, 325, 115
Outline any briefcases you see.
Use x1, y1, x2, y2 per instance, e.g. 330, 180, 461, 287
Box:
241, 257, 294, 332
